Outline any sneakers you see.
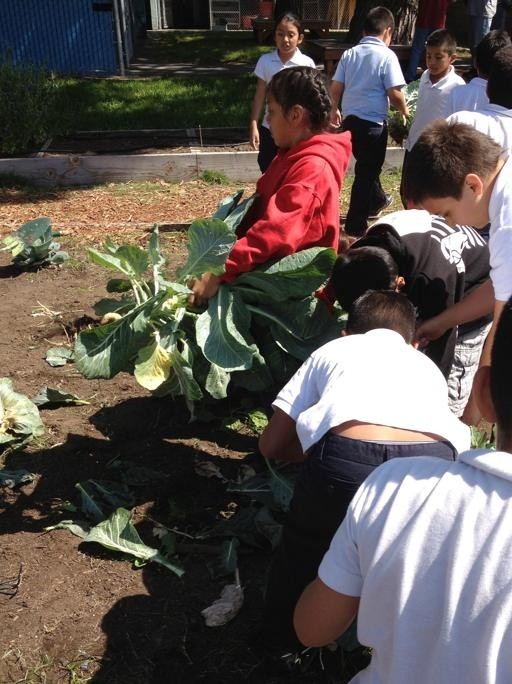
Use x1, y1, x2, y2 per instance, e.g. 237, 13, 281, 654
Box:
367, 190, 395, 219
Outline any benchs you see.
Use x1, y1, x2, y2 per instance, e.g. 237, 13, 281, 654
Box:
303, 37, 425, 75
249, 18, 329, 43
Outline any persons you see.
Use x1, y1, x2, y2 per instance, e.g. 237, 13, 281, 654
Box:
249, 11, 318, 177
443, 41, 511, 163
255, 289, 474, 637
406, 0, 450, 84
328, 6, 410, 240
463, 0, 498, 65
403, 27, 468, 193
183, 66, 356, 393
396, 117, 511, 429
291, 296, 510, 683
446, 29, 512, 117
315, 205, 495, 427
